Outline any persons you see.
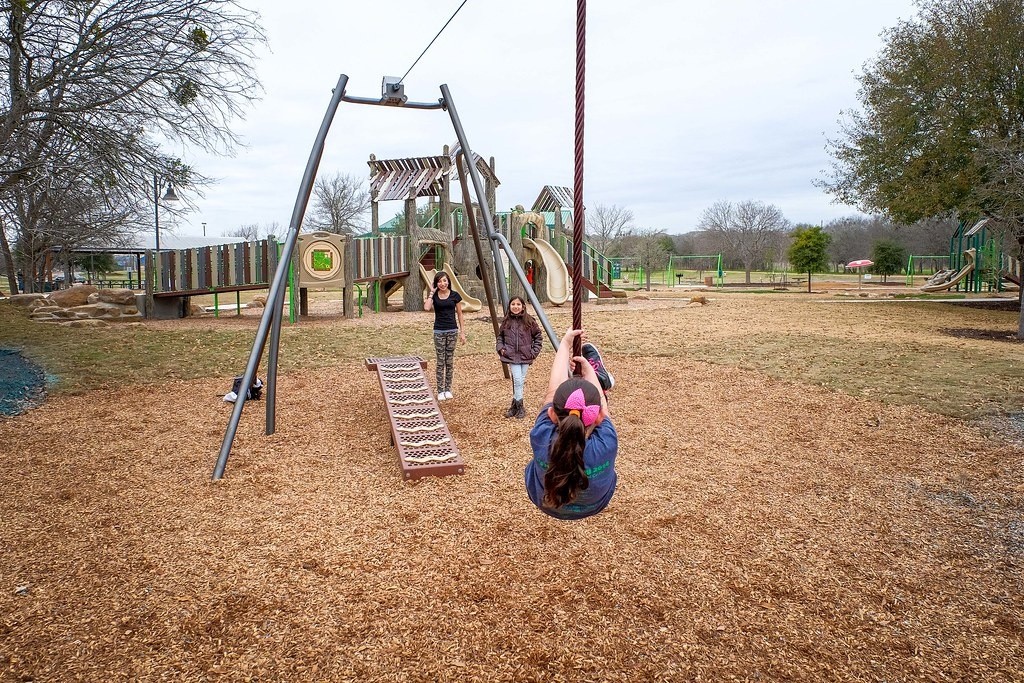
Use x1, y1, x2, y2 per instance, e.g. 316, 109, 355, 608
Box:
424, 271, 466, 400
524, 262, 534, 304
496, 296, 543, 418
525, 326, 617, 521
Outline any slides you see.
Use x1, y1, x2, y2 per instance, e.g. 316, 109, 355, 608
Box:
522, 238, 569, 305
921, 263, 1019, 291
420, 262, 482, 311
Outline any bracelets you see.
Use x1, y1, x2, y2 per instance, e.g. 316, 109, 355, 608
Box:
429, 291, 435, 299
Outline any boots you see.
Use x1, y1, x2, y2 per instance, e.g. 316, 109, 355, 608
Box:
506, 398, 517, 418
514, 399, 524, 418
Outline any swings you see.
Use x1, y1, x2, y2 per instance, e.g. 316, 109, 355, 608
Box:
570, 0, 586, 376
622, 260, 629, 283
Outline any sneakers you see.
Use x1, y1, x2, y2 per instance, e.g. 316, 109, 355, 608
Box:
438, 392, 445, 400
581, 343, 614, 394
445, 391, 454, 399
